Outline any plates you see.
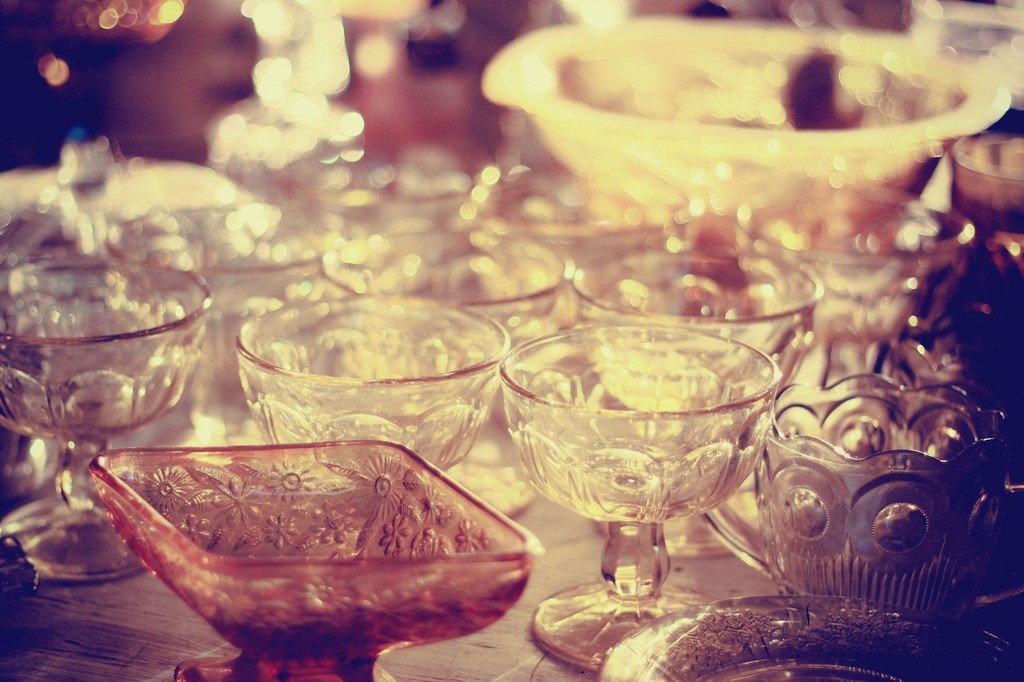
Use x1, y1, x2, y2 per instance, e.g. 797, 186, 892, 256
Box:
597, 588, 1024, 682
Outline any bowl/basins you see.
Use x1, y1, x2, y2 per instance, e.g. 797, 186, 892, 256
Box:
473, 18, 1014, 199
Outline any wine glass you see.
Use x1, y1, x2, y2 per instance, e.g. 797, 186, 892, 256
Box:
0, 132, 1024, 682
